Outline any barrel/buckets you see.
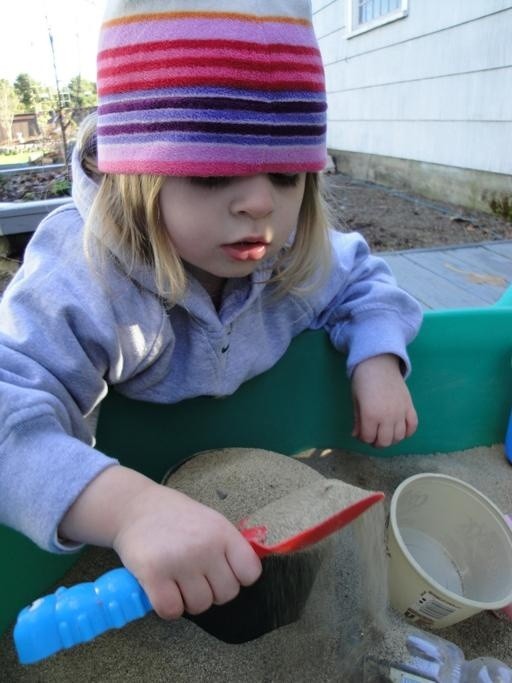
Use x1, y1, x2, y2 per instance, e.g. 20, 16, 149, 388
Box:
161, 447, 327, 645
383, 471, 512, 633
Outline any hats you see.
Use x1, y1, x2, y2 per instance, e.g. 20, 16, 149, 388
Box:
96, 1, 328, 178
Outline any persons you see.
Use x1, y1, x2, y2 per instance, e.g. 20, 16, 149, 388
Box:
2, 0, 426, 625
